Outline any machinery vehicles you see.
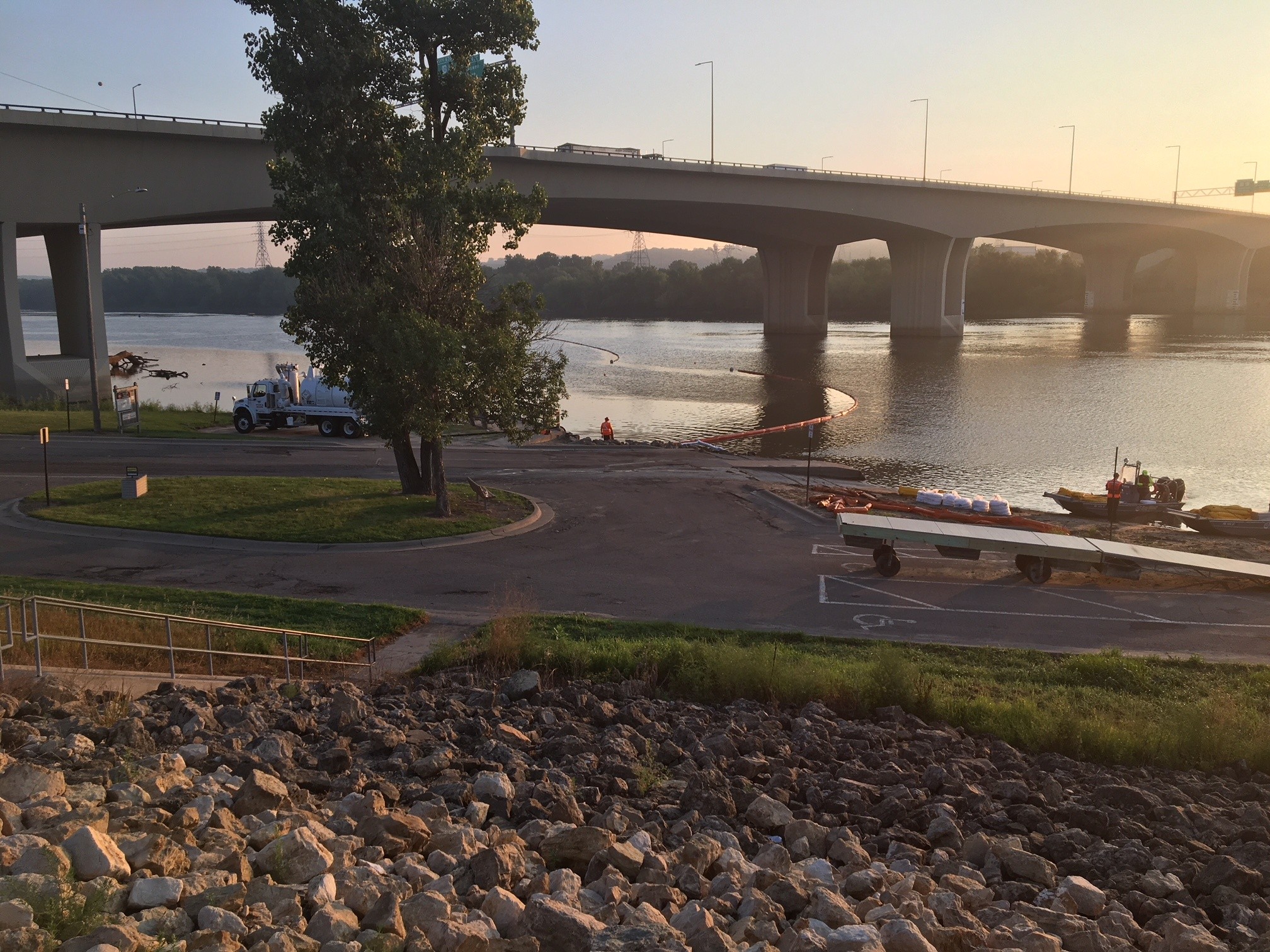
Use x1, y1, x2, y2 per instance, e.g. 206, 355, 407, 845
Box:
232, 363, 370, 438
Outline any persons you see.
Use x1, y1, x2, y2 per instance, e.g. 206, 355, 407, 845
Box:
1106, 473, 1123, 521
1151, 483, 1170, 503
1137, 470, 1154, 500
117, 393, 122, 399
601, 417, 614, 441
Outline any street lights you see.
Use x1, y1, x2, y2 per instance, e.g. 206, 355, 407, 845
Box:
1032, 181, 1043, 188
663, 139, 674, 157
1101, 190, 1111, 194
1059, 126, 1075, 194
911, 99, 928, 181
1165, 145, 1180, 204
822, 156, 833, 173
695, 61, 713, 163
1243, 162, 1257, 213
940, 169, 952, 183
133, 84, 141, 119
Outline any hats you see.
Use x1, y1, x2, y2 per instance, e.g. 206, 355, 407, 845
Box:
605, 417, 610, 420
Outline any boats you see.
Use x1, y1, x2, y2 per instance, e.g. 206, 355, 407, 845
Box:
1165, 504, 1270, 538
1042, 458, 1186, 519
